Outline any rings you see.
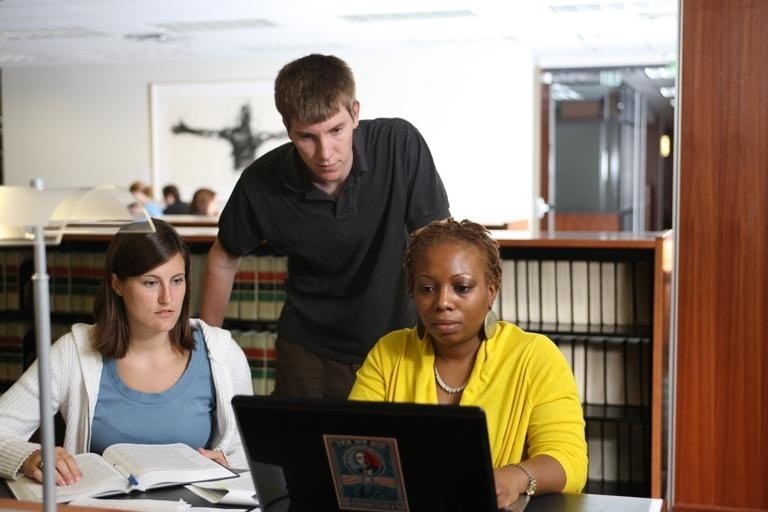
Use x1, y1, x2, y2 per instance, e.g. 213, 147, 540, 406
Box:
39, 461, 44, 469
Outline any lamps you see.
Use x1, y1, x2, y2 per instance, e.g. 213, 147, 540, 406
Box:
0, 178, 157, 512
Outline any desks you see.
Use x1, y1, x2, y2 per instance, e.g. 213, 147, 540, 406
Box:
0, 469, 665, 512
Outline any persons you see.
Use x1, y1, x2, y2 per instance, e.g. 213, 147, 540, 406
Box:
171, 105, 292, 170
159, 186, 193, 214
195, 53, 454, 404
188, 188, 219, 216
1, 216, 256, 488
346, 214, 590, 509
129, 180, 164, 218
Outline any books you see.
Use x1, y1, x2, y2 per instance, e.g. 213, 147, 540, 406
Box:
226, 251, 289, 318
5, 442, 241, 505
229, 329, 277, 396
0, 250, 107, 313
489, 258, 653, 408
586, 416, 652, 487
0, 323, 70, 380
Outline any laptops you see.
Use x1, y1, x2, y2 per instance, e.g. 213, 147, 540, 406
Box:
231, 396, 531, 512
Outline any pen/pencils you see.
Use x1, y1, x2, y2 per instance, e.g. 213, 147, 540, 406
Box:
113, 463, 140, 487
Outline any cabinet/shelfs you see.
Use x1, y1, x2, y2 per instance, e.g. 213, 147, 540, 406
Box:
0, 226, 673, 498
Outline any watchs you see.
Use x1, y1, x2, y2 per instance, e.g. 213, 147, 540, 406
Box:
507, 461, 538, 500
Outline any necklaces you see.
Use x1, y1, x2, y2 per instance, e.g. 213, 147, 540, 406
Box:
430, 362, 468, 394
